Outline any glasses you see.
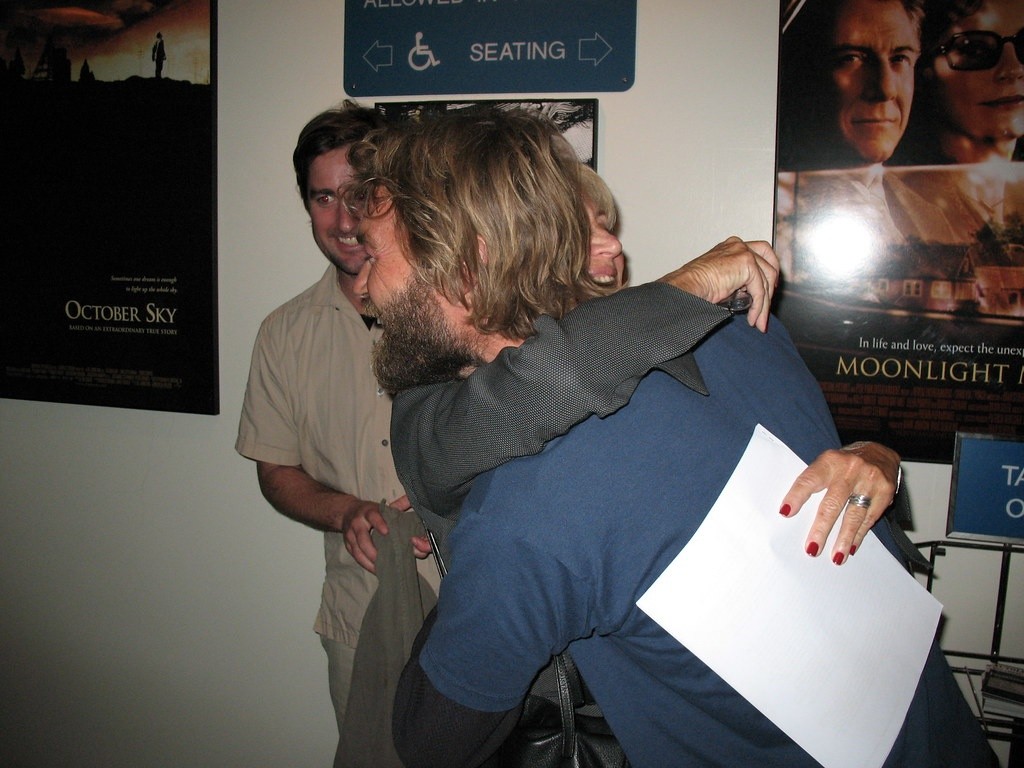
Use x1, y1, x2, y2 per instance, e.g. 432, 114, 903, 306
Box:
926, 27, 1024, 72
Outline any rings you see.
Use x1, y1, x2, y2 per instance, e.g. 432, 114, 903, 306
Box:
848, 494, 872, 510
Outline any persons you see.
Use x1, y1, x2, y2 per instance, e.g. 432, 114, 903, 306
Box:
152, 32, 167, 78
235, 100, 1002, 768
779, 1, 1024, 313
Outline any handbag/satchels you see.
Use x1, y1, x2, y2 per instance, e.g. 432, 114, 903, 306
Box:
492, 652, 629, 768
